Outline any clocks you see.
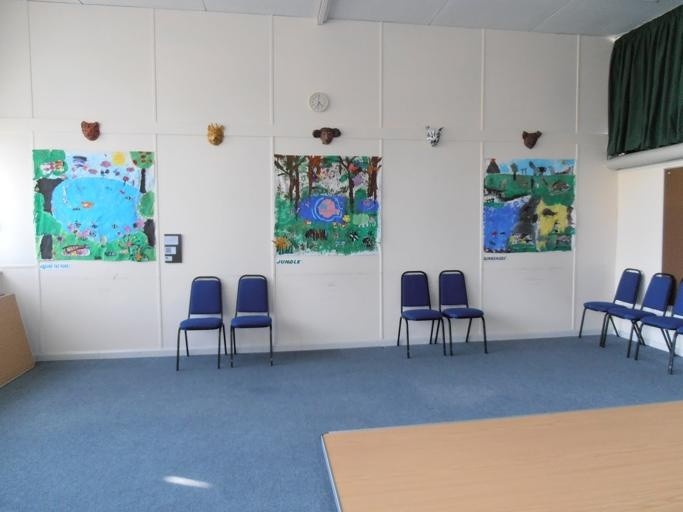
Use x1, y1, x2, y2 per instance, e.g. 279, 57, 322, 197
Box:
309, 92, 329, 113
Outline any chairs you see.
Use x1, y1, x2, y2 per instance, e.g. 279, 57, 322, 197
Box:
174, 273, 275, 370
578, 267, 683, 374
395, 270, 489, 358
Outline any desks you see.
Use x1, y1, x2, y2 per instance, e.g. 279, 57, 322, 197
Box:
319, 398, 683, 512
